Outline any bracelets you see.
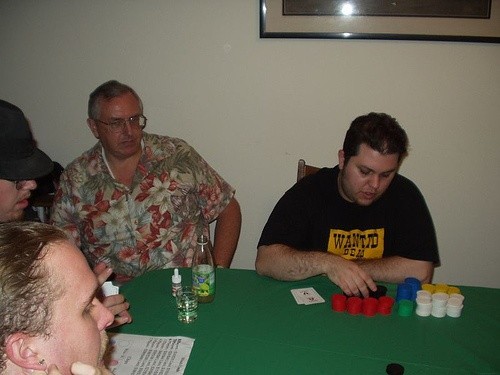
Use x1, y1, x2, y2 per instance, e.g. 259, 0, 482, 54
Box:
215, 265, 224, 269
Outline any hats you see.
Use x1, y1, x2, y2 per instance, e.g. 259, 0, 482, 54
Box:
0, 99, 56, 180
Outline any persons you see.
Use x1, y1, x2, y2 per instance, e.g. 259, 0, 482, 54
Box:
0, 83, 242, 328
0, 223, 114, 375
255, 112, 440, 297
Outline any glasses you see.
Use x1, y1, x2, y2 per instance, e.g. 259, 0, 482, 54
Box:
9, 178, 27, 191
89, 114, 147, 131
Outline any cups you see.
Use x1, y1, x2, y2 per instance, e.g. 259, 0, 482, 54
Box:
176, 287, 198, 324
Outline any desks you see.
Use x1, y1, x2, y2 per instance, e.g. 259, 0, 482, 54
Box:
91, 263, 500, 375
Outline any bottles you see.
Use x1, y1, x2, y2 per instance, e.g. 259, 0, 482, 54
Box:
190, 235, 216, 304
